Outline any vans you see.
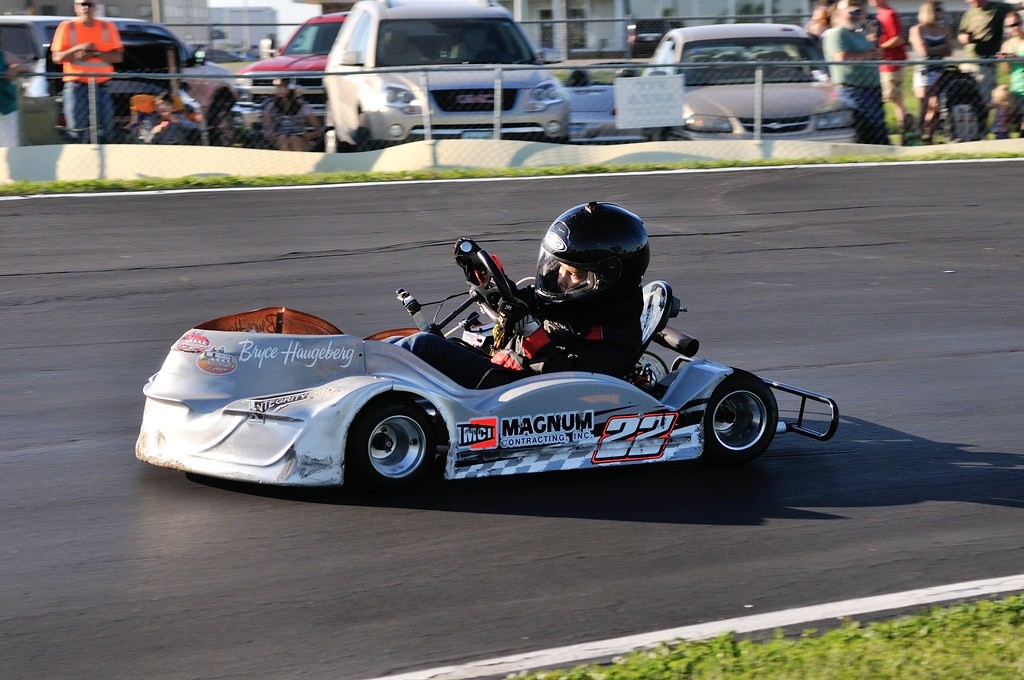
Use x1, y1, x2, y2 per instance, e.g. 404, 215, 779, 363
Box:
0, 15, 239, 137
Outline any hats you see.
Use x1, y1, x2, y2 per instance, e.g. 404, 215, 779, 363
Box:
836, 0, 865, 12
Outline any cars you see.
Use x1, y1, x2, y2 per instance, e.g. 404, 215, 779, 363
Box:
642, 23, 862, 145
562, 60, 666, 145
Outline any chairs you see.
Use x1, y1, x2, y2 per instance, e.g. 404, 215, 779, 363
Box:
456, 27, 500, 63
635, 277, 673, 368
383, 30, 427, 67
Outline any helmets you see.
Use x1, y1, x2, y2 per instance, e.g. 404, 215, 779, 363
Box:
534, 202, 650, 323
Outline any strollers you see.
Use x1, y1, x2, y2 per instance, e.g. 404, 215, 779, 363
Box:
922, 66, 988, 144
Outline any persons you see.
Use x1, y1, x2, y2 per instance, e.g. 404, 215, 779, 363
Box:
957, 0, 1024, 139
908, 0, 952, 145
261, 78, 322, 151
179, 66, 237, 148
0, 49, 33, 146
805, 0, 908, 146
50, 0, 124, 144
138, 92, 197, 145
394, 201, 651, 390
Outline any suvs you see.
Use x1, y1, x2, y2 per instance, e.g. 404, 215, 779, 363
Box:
240, 12, 356, 133
322, 1, 570, 153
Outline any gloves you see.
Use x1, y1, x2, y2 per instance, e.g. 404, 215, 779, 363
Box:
498, 297, 541, 338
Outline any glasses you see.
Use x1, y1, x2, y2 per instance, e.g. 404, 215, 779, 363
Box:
74, 2, 94, 7
935, 8, 942, 12
1009, 21, 1022, 27
